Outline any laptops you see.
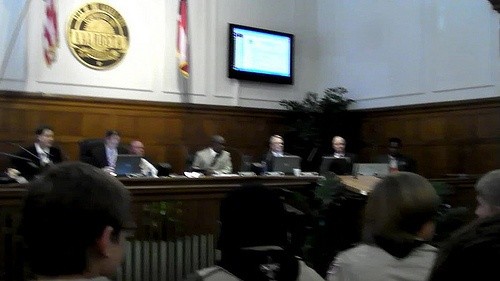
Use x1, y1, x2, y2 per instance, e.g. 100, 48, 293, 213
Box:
268, 154, 302, 173
314, 155, 352, 175
107, 154, 142, 176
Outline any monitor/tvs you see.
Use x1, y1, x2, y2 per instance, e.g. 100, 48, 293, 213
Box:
226, 22, 295, 85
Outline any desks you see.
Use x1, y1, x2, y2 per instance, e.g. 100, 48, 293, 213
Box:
0, 173, 484, 281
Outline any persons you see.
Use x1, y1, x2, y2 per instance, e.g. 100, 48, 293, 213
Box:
184, 181, 324, 281
90, 130, 153, 177
331, 136, 358, 165
15, 125, 64, 179
378, 138, 415, 174
259, 135, 292, 172
191, 135, 233, 175
430, 170, 500, 281
13, 160, 138, 281
326, 173, 440, 281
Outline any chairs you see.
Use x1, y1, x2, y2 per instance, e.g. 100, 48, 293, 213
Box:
78, 138, 104, 163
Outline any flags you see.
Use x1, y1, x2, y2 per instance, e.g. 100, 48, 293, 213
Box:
42, 0, 59, 65
174, 0, 190, 80
